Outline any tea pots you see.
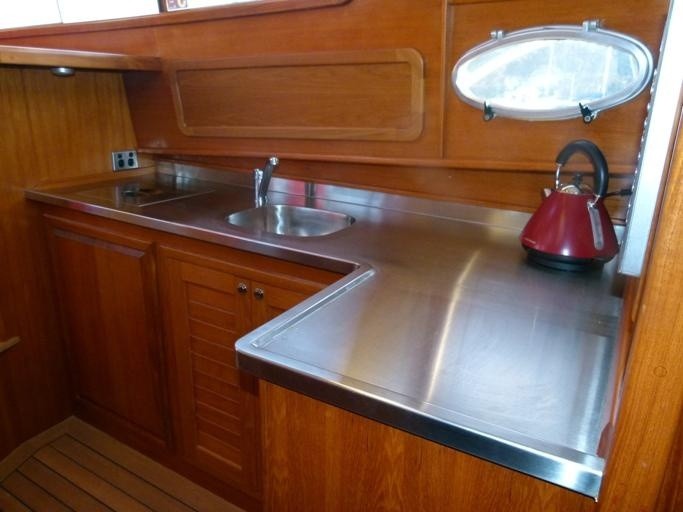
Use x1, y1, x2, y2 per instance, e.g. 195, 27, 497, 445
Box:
518, 139, 620, 277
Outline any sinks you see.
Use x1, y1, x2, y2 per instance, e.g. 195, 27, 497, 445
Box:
226, 203, 356, 240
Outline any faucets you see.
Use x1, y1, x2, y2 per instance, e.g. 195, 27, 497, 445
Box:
251, 156, 281, 207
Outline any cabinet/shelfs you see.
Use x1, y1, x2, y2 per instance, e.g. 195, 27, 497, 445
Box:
0, 46, 165, 464
44, 223, 173, 443
22, 158, 628, 511
